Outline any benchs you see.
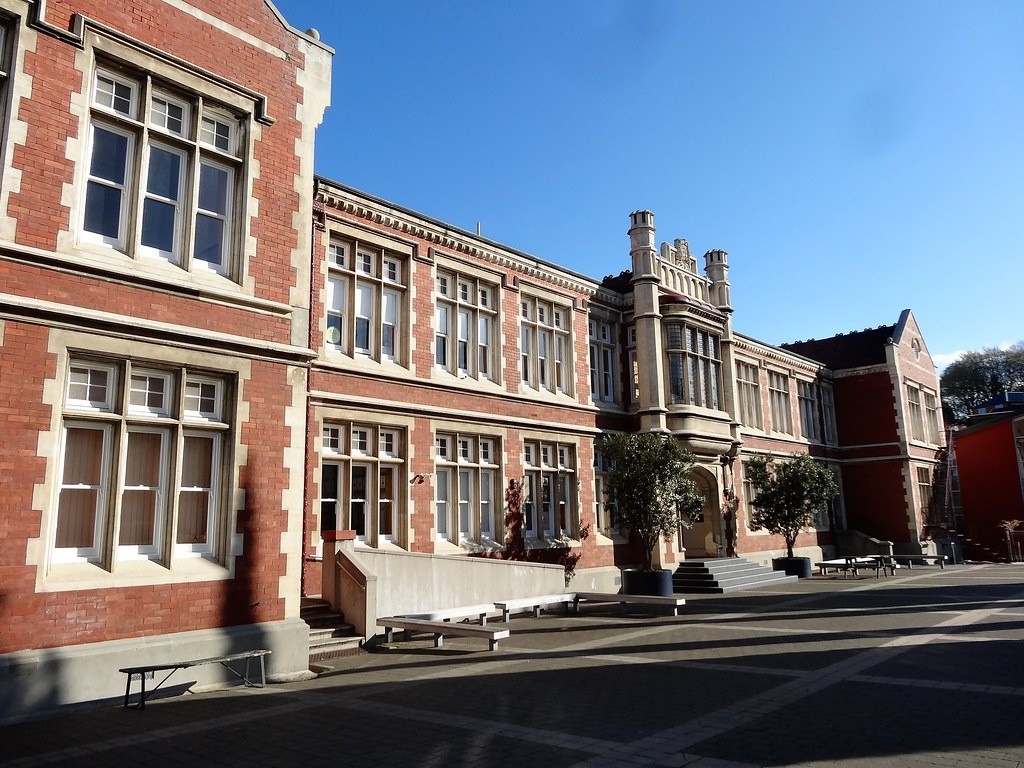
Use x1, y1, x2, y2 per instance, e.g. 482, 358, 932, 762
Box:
395, 604, 496, 639
377, 617, 509, 651
815, 555, 948, 580
571, 591, 685, 616
119, 650, 272, 710
494, 593, 576, 623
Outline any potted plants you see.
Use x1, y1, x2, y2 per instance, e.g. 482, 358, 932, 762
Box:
596, 431, 706, 596
744, 448, 838, 577
